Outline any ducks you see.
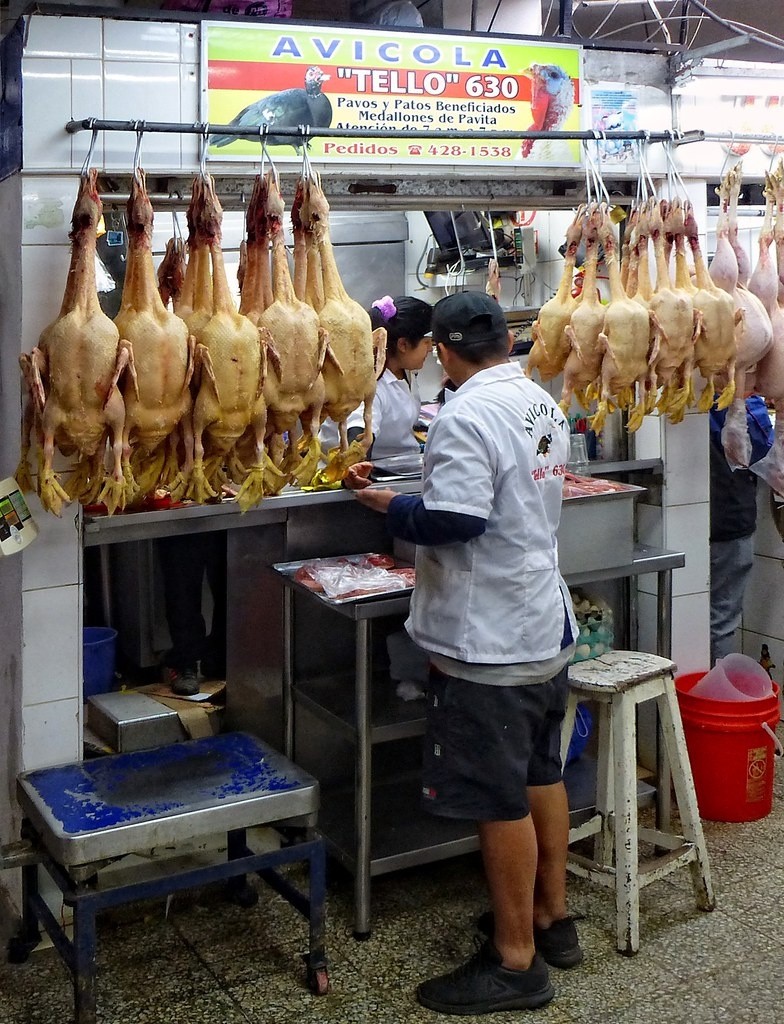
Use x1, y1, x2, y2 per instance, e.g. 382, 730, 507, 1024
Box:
17, 164, 392, 512
524, 160, 784, 494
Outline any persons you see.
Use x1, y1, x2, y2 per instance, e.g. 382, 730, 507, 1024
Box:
710, 380, 777, 670
314, 294, 439, 477
354, 290, 583, 1014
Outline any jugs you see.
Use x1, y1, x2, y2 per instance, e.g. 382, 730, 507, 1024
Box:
687, 652, 773, 701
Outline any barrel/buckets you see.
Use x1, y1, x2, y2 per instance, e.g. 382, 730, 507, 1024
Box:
673, 671, 783, 823
82, 626, 118, 704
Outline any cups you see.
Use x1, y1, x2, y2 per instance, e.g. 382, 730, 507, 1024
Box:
568, 434, 590, 471
577, 430, 596, 461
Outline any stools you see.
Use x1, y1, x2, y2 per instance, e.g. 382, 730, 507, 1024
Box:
16, 734, 329, 1024
561, 651, 715, 957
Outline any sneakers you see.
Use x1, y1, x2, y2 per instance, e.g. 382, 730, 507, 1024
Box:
479, 906, 582, 969
416, 939, 556, 1015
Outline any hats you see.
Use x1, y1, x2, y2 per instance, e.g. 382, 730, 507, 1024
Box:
433, 290, 509, 345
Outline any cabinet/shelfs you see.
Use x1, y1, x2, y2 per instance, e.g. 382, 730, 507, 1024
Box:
271, 542, 686, 938
